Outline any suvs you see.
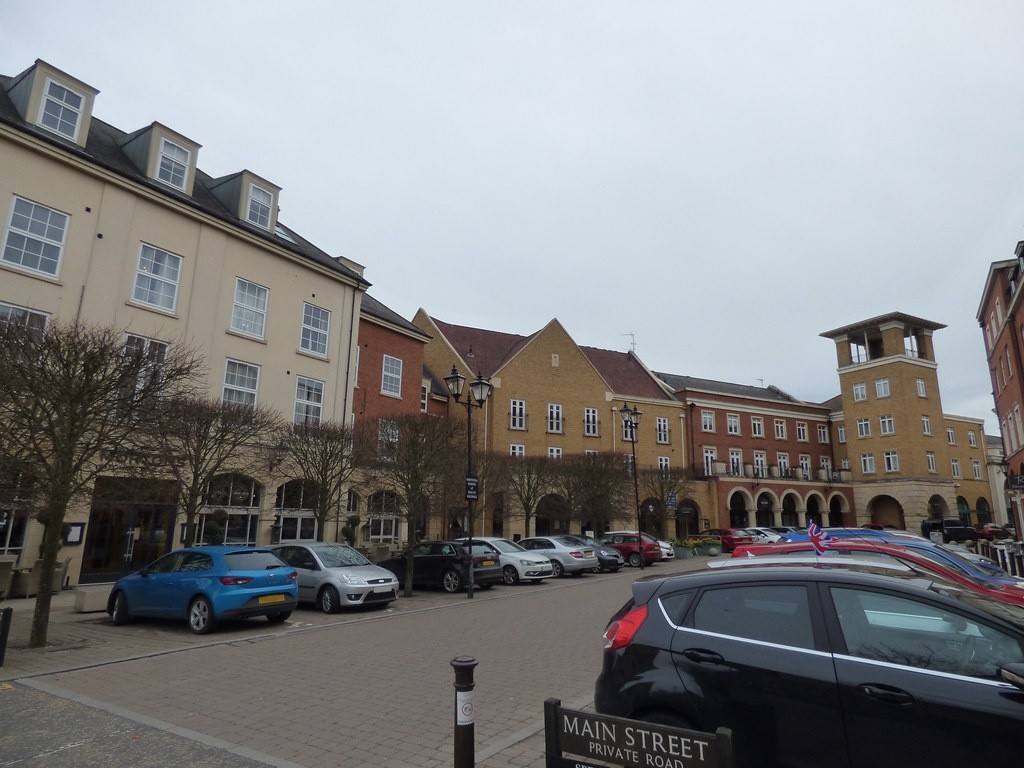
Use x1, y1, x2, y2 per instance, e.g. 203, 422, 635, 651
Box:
378, 540, 503, 593
594, 564, 1023, 768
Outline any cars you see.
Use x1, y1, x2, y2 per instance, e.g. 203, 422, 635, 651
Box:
264, 541, 400, 614
106, 545, 301, 636
456, 510, 1024, 636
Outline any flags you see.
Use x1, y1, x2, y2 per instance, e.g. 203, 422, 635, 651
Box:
809, 523, 839, 556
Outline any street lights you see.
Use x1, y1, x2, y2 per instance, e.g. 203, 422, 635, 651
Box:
619, 401, 643, 569
444, 362, 491, 598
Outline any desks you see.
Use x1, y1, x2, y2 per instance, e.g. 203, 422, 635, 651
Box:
11, 566, 33, 573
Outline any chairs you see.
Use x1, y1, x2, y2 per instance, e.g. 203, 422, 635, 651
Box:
12, 559, 43, 598
51, 556, 71, 594
354, 540, 408, 561
0, 560, 15, 601
0, 555, 19, 567
